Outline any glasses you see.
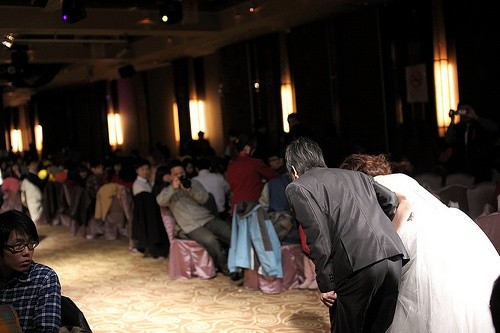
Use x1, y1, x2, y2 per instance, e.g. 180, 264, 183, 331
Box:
0, 238, 39, 254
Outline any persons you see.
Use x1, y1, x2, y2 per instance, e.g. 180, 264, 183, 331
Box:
0, 121, 290, 285
283, 136, 410, 333
285, 112, 327, 144
0, 209, 61, 333
443, 99, 496, 181
432, 140, 469, 177
337, 152, 500, 333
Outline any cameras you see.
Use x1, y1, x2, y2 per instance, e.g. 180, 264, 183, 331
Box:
178, 175, 191, 188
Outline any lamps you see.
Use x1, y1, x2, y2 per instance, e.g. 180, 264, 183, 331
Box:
160, 0, 182, 25
280, 53, 297, 132
188, 78, 206, 140
62, 0, 87, 24
434, 26, 461, 136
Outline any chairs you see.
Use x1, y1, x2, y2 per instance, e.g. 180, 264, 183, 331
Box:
0, 161, 500, 333
